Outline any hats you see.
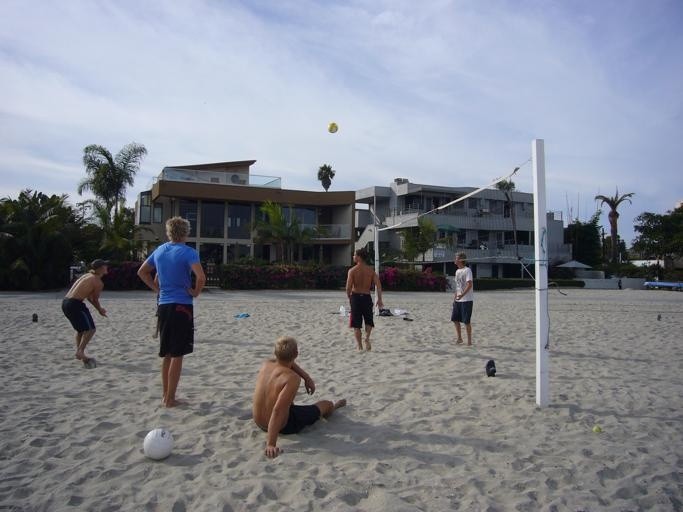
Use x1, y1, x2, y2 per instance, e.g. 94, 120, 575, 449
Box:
90, 259, 109, 269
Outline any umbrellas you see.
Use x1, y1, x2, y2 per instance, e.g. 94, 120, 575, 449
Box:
554, 260, 592, 269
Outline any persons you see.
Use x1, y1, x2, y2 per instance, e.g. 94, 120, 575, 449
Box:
249, 335, 346, 459
448, 252, 474, 347
61, 256, 108, 361
150, 273, 160, 339
345, 250, 383, 351
135, 213, 205, 408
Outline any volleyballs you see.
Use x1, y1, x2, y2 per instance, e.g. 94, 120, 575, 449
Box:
143, 428, 175, 459
327, 122, 338, 133
